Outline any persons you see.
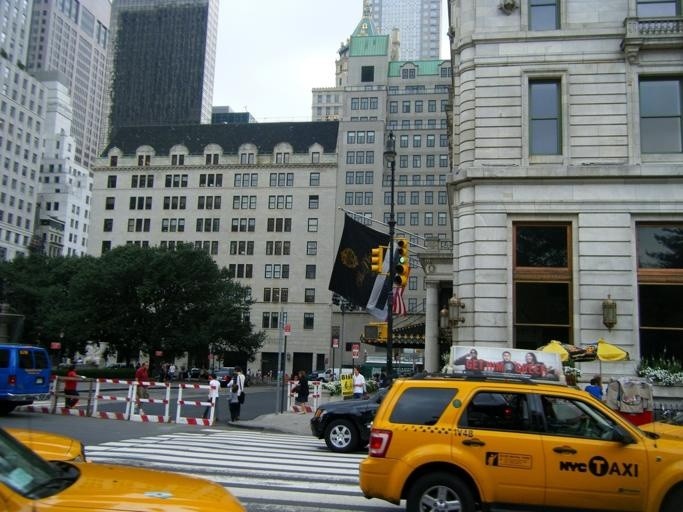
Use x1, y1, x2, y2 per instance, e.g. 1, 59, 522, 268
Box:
585, 379, 603, 400
453, 348, 488, 371
540, 396, 586, 433
493, 351, 521, 374
64, 355, 366, 421
520, 352, 547, 377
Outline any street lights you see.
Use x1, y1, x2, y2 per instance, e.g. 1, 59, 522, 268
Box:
381, 131, 397, 387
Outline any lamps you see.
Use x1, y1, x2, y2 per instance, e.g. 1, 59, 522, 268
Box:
441, 304, 448, 330
602, 294, 617, 332
448, 294, 465, 325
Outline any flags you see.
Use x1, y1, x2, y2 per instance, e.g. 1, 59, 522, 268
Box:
327, 211, 411, 321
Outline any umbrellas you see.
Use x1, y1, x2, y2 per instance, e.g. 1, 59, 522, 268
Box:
572, 336, 629, 378
536, 339, 587, 364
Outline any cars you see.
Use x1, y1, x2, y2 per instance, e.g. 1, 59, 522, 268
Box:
69, 356, 244, 387
308, 370, 321, 382
0, 426, 248, 512
0, 427, 87, 465
309, 380, 513, 451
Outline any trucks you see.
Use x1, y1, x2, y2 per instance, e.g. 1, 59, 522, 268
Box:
317, 368, 353, 382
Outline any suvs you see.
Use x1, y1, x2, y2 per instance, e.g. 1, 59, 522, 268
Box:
1, 343, 57, 417
356, 373, 683, 511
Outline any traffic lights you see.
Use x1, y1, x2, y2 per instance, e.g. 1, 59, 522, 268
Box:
393, 237, 409, 286
370, 246, 382, 273
